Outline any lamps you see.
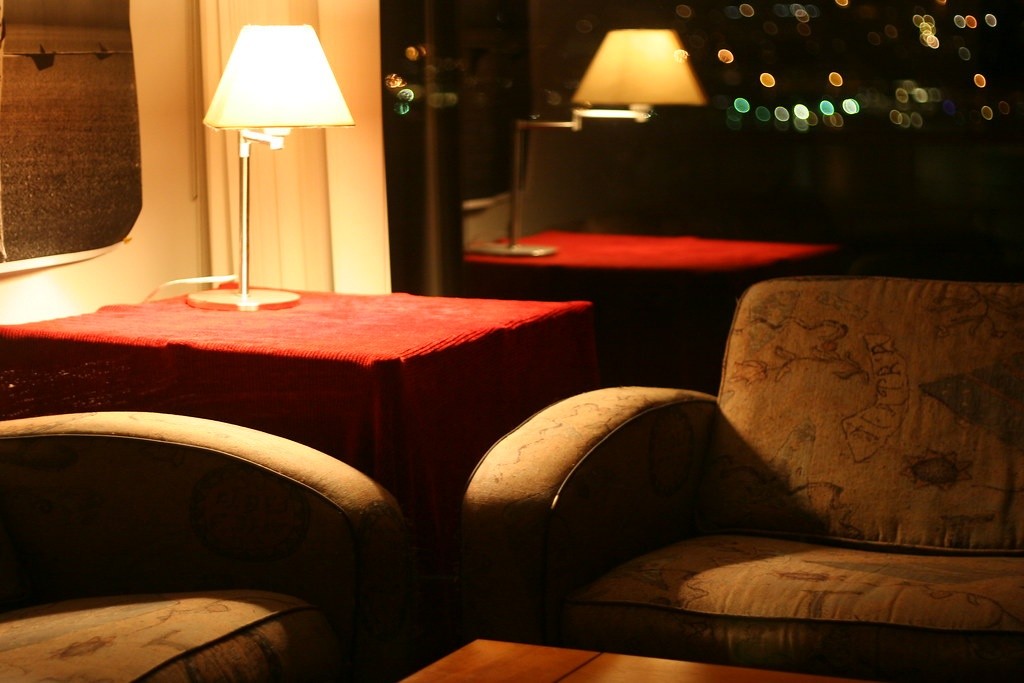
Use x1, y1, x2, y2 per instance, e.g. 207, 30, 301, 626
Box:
465, 29, 705, 256
188, 24, 356, 312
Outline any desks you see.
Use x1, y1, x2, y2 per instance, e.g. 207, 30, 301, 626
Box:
1, 286, 603, 668
466, 232, 838, 395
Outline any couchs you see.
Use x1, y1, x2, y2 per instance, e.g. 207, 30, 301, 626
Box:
457, 278, 1024, 683
0, 413, 413, 683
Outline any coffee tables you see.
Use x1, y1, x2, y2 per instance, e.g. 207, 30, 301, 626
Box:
397, 640, 878, 683
